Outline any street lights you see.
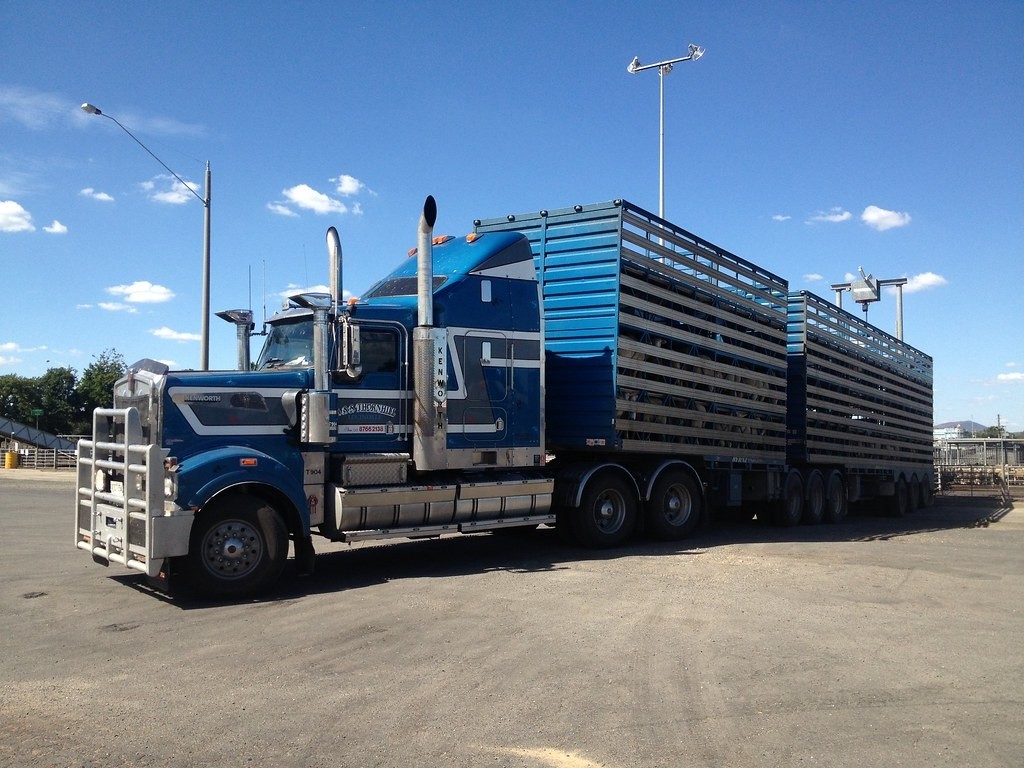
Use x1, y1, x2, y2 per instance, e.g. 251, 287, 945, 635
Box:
626, 44, 706, 273
80, 102, 212, 371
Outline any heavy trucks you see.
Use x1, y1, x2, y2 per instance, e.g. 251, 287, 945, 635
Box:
74, 192, 934, 605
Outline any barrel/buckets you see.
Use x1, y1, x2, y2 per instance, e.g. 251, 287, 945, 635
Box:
4, 452, 18, 469
